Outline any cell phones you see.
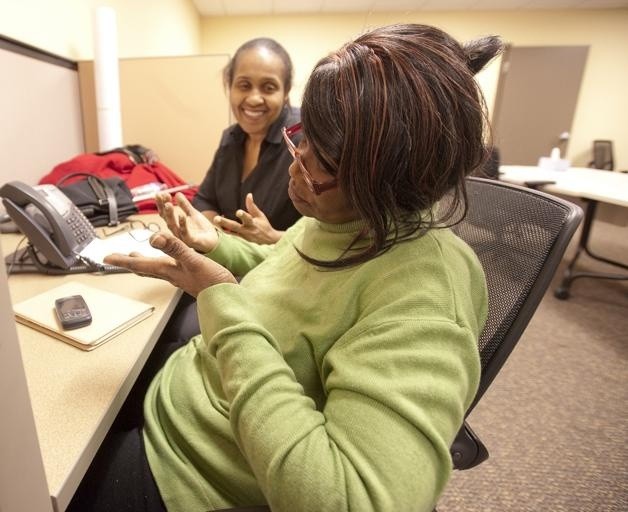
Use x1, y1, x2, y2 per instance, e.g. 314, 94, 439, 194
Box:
55, 294, 92, 330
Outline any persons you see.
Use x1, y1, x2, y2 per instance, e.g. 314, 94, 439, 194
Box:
90, 20, 505, 512
149, 37, 303, 363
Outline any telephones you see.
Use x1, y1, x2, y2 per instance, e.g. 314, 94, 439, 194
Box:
0, 181, 97, 270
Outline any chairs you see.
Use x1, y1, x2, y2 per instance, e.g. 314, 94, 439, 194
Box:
435, 145, 583, 469
587, 140, 627, 172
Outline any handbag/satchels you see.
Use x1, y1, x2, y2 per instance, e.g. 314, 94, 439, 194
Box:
55, 170, 137, 230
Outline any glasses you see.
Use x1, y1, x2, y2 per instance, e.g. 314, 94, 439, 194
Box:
102, 219, 162, 244
278, 117, 357, 198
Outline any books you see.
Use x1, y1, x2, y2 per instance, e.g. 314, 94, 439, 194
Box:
12, 282, 155, 350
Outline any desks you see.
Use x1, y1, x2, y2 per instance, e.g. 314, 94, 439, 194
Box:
496, 163, 628, 298
1, 213, 194, 511
